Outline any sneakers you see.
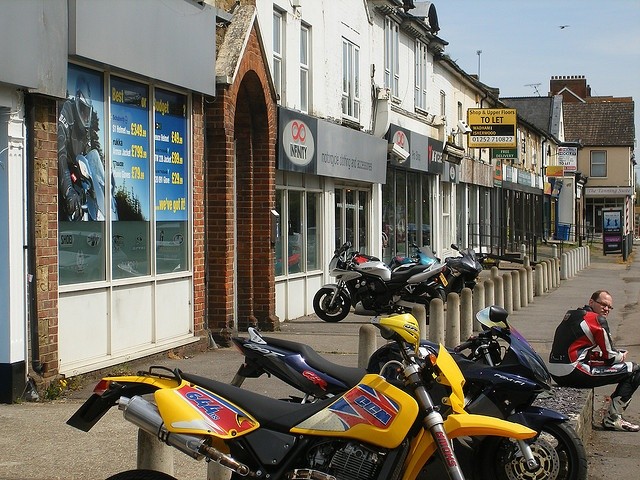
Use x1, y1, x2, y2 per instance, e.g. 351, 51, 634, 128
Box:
602, 413, 640, 432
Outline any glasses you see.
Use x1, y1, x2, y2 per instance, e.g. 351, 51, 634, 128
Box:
594, 300, 614, 311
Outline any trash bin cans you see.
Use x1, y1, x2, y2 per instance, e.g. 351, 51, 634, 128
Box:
556, 224, 569, 240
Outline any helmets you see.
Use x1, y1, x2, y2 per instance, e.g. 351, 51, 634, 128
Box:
73, 75, 93, 136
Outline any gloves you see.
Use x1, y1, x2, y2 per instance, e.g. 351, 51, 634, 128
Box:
65, 190, 82, 218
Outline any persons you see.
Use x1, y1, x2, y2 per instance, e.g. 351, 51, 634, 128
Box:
547, 290, 640, 433
58, 58, 113, 226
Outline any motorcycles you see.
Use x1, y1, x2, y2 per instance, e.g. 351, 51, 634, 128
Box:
314, 240, 448, 325
436, 243, 484, 307
66, 311, 538, 480
388, 243, 439, 271
230, 306, 587, 480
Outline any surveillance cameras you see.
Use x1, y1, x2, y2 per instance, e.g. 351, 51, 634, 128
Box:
386, 142, 410, 164
452, 120, 473, 138
